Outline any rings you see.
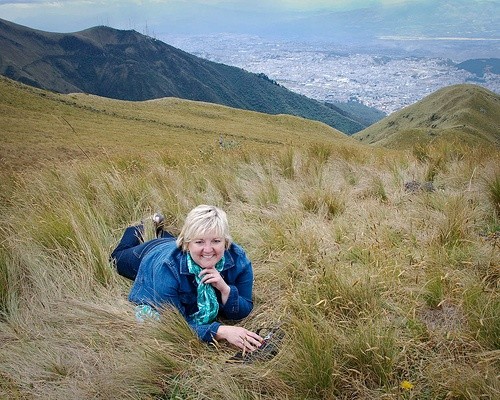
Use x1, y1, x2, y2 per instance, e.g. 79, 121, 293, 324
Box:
242, 336, 247, 341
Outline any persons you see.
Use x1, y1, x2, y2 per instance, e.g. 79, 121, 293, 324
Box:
108, 205, 263, 352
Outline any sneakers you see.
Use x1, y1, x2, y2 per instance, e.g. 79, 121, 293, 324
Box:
142, 213, 163, 225
